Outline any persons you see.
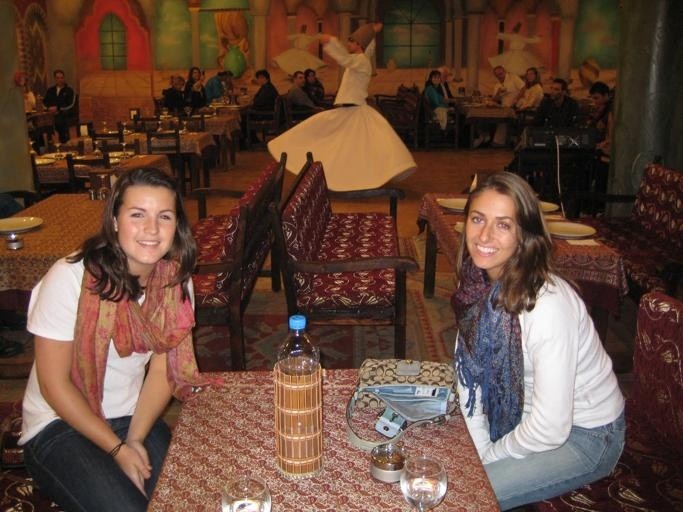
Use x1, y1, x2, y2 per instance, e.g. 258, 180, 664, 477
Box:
41, 69, 76, 143
272, 25, 329, 78
18, 165, 195, 512
266, 30, 417, 193
15, 70, 45, 156
423, 66, 614, 163
454, 170, 626, 512
162, 67, 334, 152
487, 23, 540, 74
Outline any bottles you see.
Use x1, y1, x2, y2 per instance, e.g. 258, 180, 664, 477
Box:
273, 314, 321, 478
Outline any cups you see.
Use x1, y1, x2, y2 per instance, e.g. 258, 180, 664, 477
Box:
370, 442, 409, 483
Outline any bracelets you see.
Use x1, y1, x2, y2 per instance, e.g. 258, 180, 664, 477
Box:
109, 441, 126, 458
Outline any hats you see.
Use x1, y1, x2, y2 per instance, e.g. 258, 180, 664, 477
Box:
349, 23, 377, 53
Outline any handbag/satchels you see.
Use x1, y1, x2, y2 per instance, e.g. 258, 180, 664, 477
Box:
345, 357, 458, 446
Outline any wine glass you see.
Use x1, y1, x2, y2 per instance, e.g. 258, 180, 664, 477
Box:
221, 474, 272, 512
400, 454, 448, 512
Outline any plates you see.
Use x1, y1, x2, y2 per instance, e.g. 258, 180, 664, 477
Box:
35, 151, 132, 169
455, 219, 469, 231
438, 194, 475, 213
539, 201, 559, 215
1, 216, 43, 235
544, 222, 594, 241
